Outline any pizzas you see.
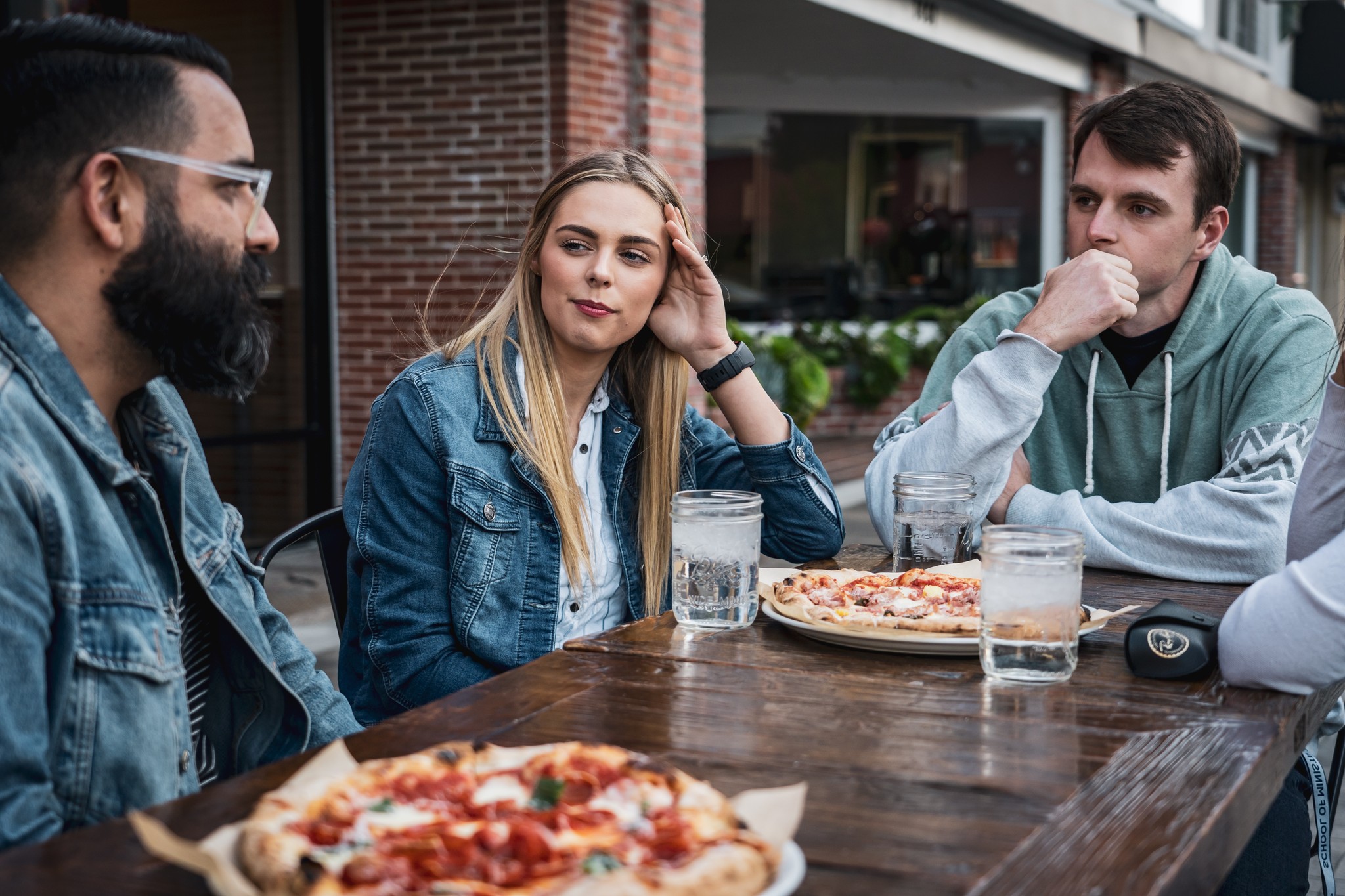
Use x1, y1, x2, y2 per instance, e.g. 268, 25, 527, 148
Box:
770, 567, 1092, 638
232, 739, 780, 896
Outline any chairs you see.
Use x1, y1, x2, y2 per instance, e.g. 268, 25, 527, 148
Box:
253, 506, 349, 642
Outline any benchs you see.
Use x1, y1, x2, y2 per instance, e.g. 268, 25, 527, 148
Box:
761, 260, 931, 320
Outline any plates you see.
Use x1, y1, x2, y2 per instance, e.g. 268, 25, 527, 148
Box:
761, 599, 1108, 655
199, 820, 807, 896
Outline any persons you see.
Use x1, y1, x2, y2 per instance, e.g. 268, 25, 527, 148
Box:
0, 12, 367, 855
862, 81, 1345, 896
335, 147, 849, 728
1217, 337, 1345, 696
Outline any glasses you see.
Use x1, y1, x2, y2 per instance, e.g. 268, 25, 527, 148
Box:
107, 145, 272, 236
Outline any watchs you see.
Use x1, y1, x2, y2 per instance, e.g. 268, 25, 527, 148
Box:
696, 340, 756, 393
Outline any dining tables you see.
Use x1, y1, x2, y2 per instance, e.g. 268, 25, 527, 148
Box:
0, 546, 1345, 896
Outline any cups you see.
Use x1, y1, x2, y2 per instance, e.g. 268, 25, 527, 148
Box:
978, 524, 1087, 686
670, 490, 764, 632
892, 471, 977, 573
